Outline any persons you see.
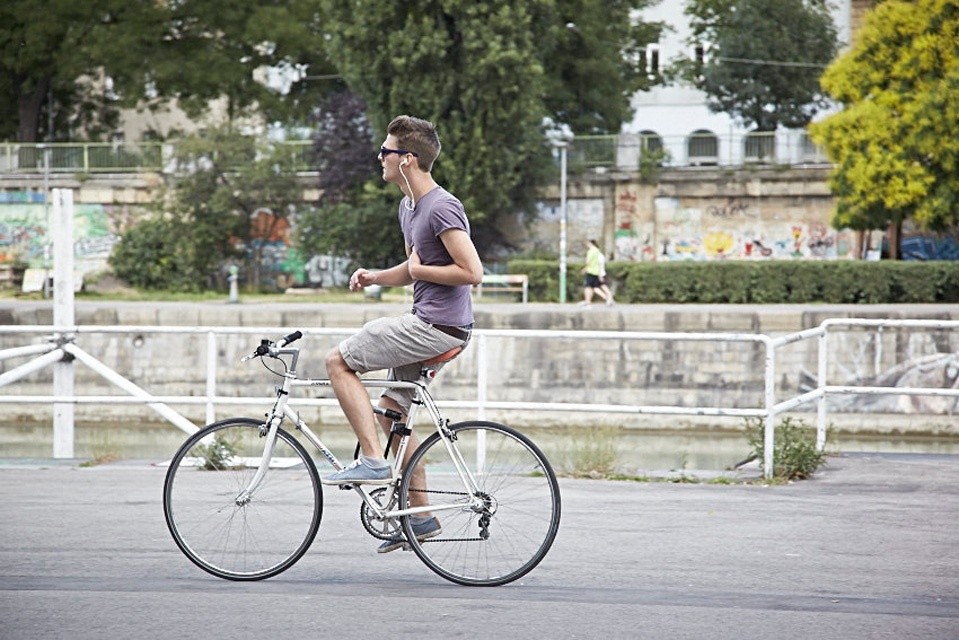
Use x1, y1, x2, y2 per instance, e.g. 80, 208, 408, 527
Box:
319, 114, 483, 555
576, 239, 615, 308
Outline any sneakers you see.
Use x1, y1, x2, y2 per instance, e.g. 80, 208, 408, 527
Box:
320, 453, 393, 485
378, 514, 442, 553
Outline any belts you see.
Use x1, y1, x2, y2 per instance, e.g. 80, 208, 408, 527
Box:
433, 321, 472, 342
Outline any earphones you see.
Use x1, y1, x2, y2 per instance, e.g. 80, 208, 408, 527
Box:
401, 158, 408, 165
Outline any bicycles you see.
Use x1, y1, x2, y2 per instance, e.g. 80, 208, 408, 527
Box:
163, 330, 561, 587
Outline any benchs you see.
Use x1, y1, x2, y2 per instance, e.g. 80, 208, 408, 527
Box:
470, 274, 528, 303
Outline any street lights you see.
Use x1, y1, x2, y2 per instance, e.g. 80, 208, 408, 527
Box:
542, 116, 575, 304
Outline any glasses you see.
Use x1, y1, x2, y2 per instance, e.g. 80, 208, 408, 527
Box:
381, 144, 418, 160
